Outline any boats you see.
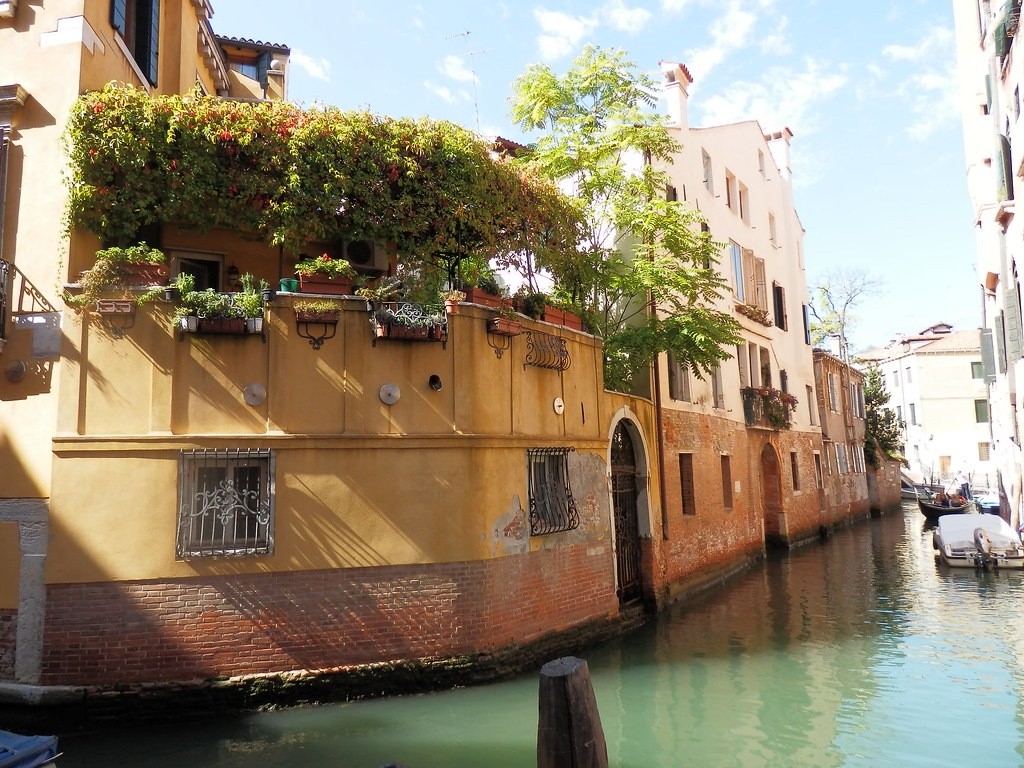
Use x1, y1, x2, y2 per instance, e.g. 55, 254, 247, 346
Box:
975, 495, 1000, 514
932, 513, 1024, 570
901, 459, 974, 521
901, 488, 940, 500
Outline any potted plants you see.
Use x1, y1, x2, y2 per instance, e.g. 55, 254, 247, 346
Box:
373, 266, 582, 338
292, 301, 341, 323
59, 242, 269, 335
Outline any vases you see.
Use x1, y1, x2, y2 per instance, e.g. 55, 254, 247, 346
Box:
300, 273, 352, 295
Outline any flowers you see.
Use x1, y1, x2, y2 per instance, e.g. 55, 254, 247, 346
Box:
295, 252, 358, 281
